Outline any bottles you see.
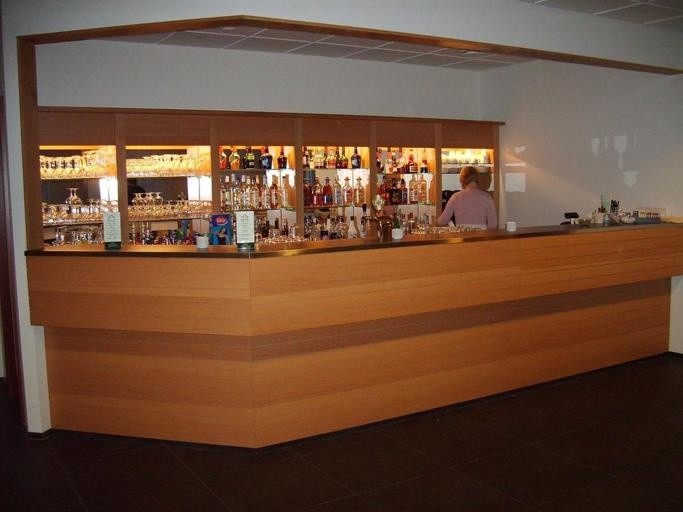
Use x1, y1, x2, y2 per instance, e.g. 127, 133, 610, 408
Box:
593, 205, 659, 224
441, 150, 490, 172
217, 145, 431, 243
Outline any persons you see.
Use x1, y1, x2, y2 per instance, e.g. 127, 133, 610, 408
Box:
436, 165, 497, 230
213, 227, 230, 245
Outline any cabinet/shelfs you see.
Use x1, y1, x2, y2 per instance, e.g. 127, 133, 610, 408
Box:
36, 105, 505, 245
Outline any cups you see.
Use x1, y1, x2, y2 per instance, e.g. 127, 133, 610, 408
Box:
506, 222, 516, 232
195, 236, 209, 249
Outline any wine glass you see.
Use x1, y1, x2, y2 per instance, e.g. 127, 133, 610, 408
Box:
37, 149, 213, 244
